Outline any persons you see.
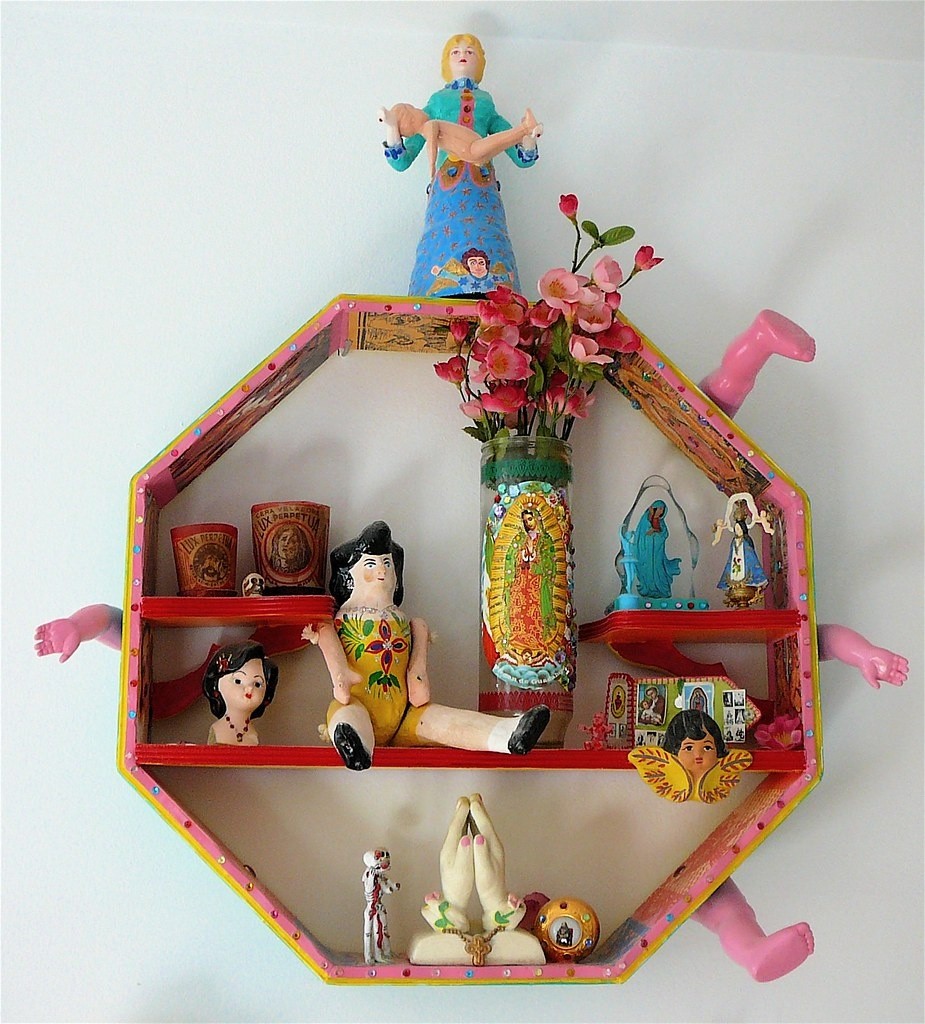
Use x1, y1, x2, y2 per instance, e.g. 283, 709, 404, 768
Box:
621, 312, 910, 981
318, 520, 550, 771
379, 32, 543, 299
422, 793, 525, 933
35, 604, 124, 663
201, 640, 280, 746
361, 849, 402, 965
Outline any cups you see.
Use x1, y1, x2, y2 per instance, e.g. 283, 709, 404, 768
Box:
251, 501, 330, 595
171, 523, 239, 597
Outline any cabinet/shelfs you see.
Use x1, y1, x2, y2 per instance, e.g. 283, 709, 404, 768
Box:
120, 291, 826, 988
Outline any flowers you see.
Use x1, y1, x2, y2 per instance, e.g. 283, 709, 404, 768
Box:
433, 192, 662, 443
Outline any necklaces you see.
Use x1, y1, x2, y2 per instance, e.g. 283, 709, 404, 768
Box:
337, 604, 397, 620
226, 712, 250, 743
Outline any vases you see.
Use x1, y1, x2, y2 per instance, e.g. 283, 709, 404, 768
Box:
478, 433, 580, 743
